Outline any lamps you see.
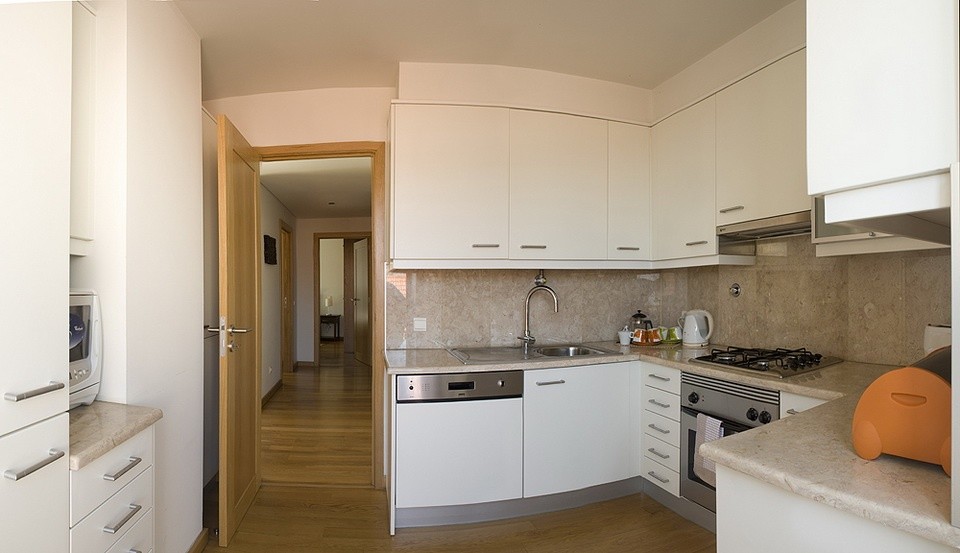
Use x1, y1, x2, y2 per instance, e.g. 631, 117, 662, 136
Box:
325, 295, 334, 315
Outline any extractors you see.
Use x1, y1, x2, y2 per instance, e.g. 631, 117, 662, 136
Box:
716, 211, 811, 238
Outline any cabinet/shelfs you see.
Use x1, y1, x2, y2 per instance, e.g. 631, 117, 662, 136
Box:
517, 364, 679, 499
0, 0, 205, 553
388, 44, 960, 273
779, 390, 829, 419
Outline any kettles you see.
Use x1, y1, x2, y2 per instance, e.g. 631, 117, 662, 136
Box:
682, 309, 714, 346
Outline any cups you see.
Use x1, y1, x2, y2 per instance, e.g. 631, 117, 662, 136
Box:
618, 332, 630, 345
647, 328, 662, 343
630, 328, 647, 343
658, 326, 668, 340
669, 326, 682, 340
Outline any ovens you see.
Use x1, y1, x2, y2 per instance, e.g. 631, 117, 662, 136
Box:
680, 372, 779, 513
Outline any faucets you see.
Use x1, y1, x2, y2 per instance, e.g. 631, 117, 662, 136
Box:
517, 285, 559, 351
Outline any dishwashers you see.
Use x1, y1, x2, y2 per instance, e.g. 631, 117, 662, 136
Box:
395, 371, 523, 509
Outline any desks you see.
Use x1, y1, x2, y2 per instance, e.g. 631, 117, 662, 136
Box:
320, 315, 341, 341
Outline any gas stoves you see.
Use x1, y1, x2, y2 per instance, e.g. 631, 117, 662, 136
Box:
681, 346, 843, 427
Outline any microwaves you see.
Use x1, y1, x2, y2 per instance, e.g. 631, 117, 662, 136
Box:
69, 289, 102, 409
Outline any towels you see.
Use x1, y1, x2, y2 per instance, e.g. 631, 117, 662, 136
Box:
693, 413, 724, 489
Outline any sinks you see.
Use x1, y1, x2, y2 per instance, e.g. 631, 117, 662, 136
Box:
534, 347, 607, 358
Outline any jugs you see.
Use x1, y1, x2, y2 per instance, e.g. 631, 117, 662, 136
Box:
631, 310, 653, 331
